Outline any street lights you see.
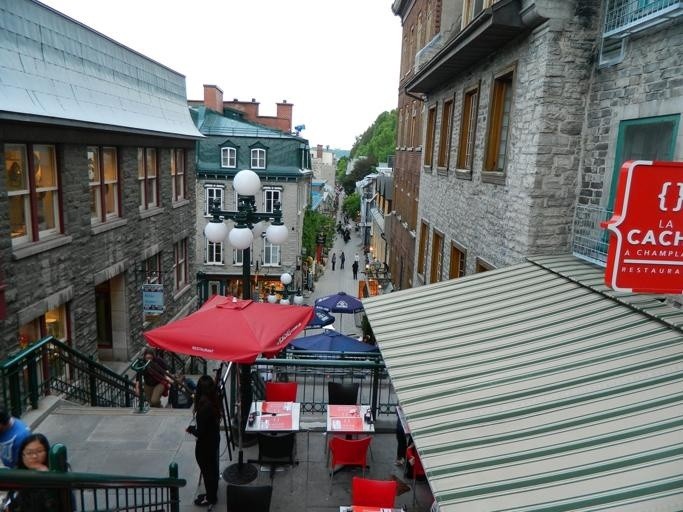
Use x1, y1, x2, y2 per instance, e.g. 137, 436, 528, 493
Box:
267, 272, 303, 304
203, 169, 289, 448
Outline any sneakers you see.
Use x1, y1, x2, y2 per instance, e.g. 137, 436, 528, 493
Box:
194, 494, 217, 506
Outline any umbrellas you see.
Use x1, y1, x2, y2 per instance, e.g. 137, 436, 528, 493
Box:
315, 292, 364, 332
287, 328, 379, 352
298, 303, 336, 336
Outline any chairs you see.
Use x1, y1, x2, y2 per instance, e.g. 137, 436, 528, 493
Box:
225, 379, 424, 512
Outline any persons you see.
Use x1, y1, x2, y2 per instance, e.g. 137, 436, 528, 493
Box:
332, 183, 389, 280
261, 287, 269, 303
135, 349, 170, 408
167, 369, 195, 408
0, 410, 75, 512
186, 375, 223, 507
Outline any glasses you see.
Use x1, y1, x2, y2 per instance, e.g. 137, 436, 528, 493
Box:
22, 449, 47, 458
